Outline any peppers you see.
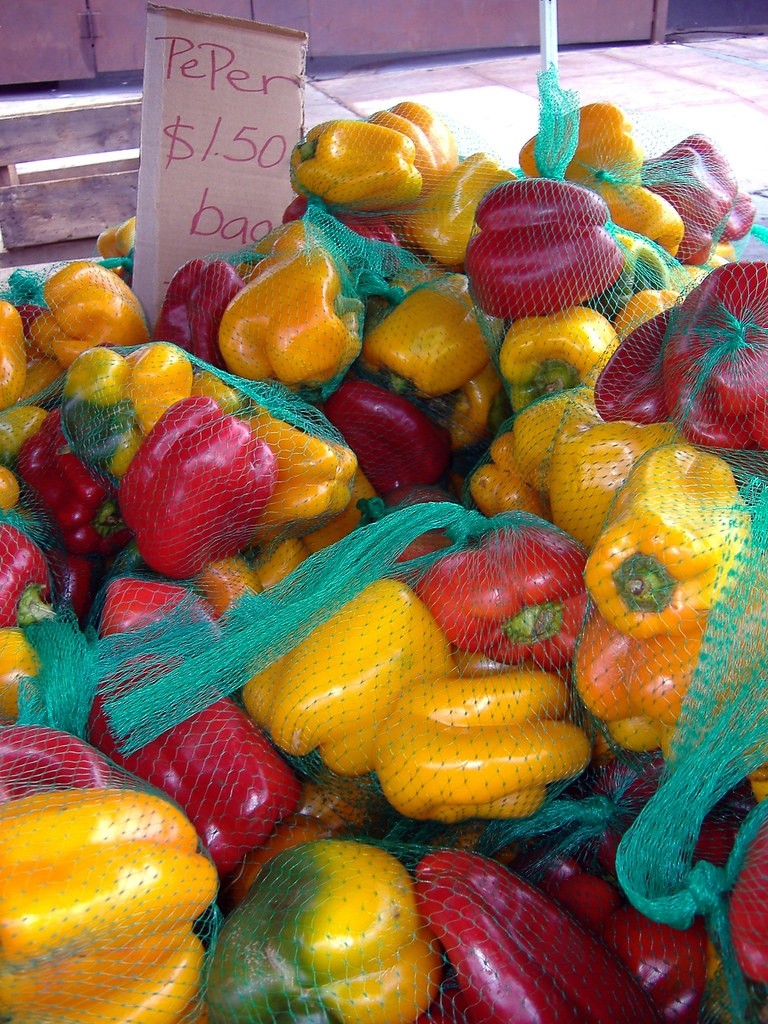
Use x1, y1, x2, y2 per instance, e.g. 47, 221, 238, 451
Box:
0, 97, 768, 1024
